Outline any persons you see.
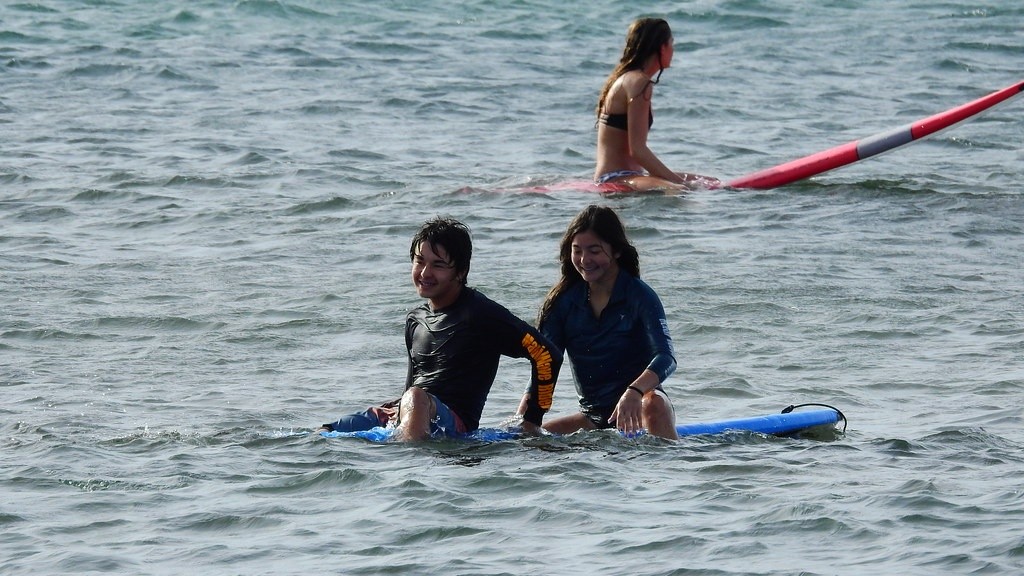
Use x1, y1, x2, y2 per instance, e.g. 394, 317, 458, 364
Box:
314, 217, 565, 438
513, 205, 680, 441
596, 17, 720, 191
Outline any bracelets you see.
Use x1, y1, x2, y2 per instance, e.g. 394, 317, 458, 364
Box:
626, 385, 645, 397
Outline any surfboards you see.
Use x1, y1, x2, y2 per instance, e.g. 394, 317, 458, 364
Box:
449, 79, 1024, 198
281, 407, 845, 453
